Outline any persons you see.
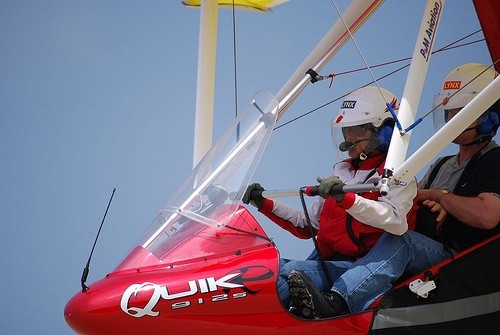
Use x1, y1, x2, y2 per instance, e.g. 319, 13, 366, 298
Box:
287, 62, 500, 322
243, 86, 418, 308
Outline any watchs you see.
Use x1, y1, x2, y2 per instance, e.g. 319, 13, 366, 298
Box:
435, 189, 448, 203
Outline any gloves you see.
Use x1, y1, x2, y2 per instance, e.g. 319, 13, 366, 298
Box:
242, 182, 265, 209
317, 174, 345, 202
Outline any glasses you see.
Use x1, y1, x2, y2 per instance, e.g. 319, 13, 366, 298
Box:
343, 126, 377, 137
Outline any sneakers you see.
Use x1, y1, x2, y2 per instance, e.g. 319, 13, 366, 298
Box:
287, 269, 342, 319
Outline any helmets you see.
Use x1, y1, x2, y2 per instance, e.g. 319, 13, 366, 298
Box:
331, 86, 400, 160
433, 63, 500, 133
